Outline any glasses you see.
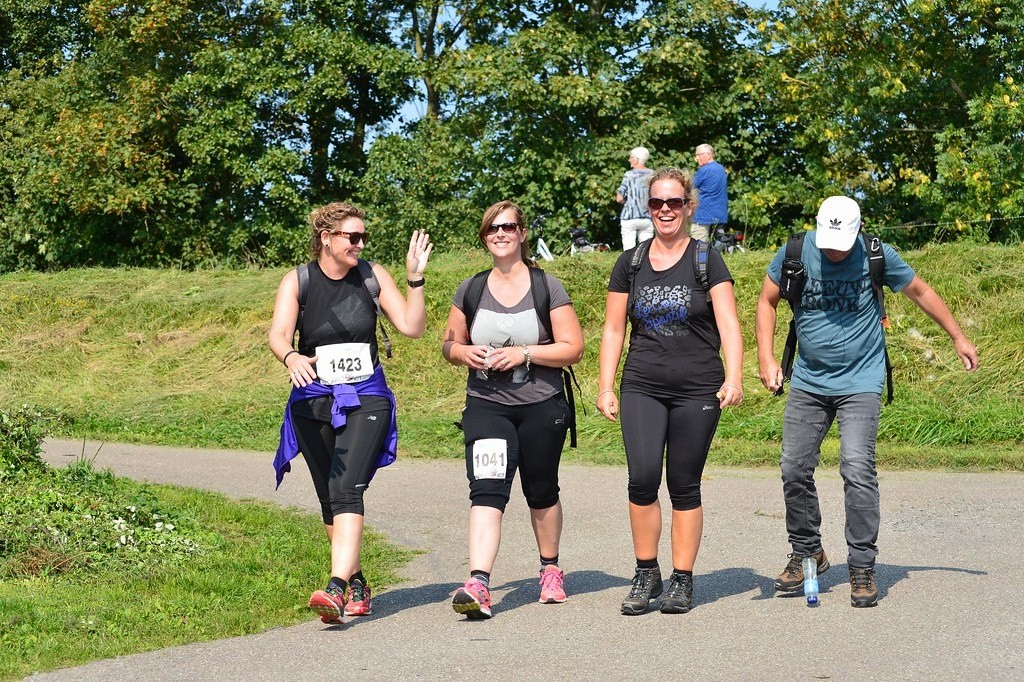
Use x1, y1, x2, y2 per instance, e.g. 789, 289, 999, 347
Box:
485, 222, 523, 235
328, 231, 370, 244
648, 197, 691, 211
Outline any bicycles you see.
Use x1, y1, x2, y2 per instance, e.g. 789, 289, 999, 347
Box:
709, 223, 746, 256
529, 211, 612, 264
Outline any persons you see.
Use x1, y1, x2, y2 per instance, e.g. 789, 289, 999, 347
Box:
615, 147, 658, 252
441, 202, 585, 621
755, 196, 977, 608
595, 169, 743, 610
269, 203, 433, 625
689, 143, 729, 244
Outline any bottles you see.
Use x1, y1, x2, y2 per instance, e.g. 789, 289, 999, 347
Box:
803, 558, 819, 605
528, 256, 539, 268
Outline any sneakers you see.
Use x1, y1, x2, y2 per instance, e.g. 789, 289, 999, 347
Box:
538, 563, 566, 605
848, 564, 878, 608
621, 567, 664, 614
659, 572, 693, 613
343, 584, 371, 616
774, 547, 830, 591
309, 587, 346, 624
448, 577, 492, 620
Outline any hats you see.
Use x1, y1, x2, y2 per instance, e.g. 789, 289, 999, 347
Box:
815, 196, 861, 253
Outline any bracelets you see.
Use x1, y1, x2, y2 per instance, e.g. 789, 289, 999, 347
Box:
719, 384, 743, 397
407, 276, 425, 287
596, 390, 616, 401
283, 350, 298, 368
521, 344, 531, 370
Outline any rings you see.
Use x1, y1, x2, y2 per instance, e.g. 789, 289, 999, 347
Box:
504, 359, 508, 364
294, 371, 299, 375
